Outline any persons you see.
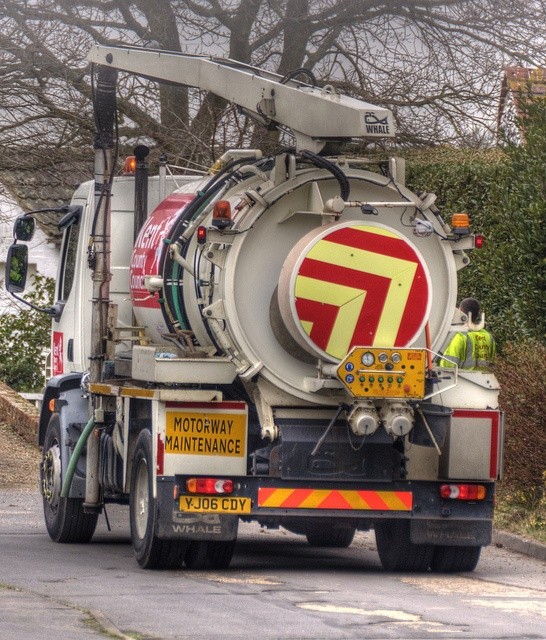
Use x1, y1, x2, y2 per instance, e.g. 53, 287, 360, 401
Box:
439, 298, 495, 372
9, 249, 24, 283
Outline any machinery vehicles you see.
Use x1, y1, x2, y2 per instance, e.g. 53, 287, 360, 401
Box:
3, 44, 506, 577
